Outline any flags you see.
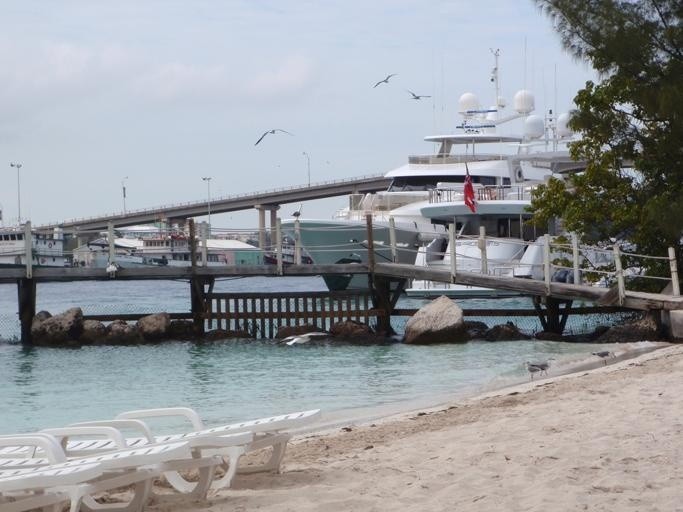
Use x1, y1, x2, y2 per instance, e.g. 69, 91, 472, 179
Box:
463, 175, 477, 213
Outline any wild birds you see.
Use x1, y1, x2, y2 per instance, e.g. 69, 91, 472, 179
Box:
405, 90, 432, 100
272, 330, 333, 346
254, 128, 291, 146
590, 350, 619, 366
526, 363, 548, 380
373, 74, 397, 89
